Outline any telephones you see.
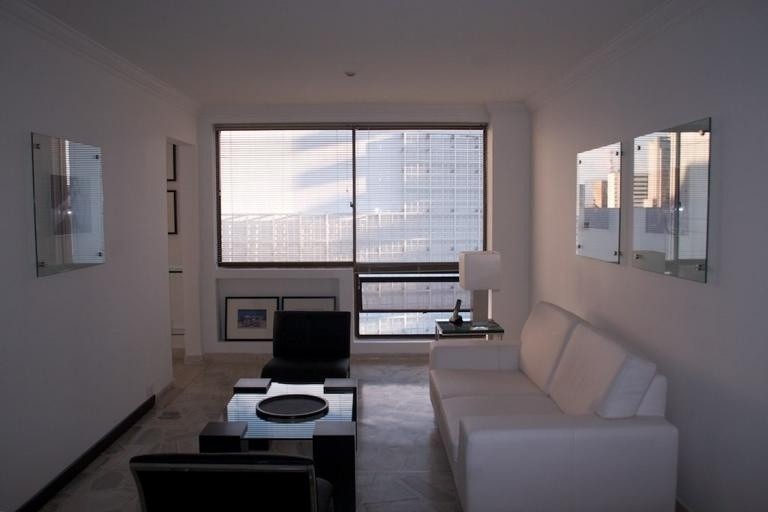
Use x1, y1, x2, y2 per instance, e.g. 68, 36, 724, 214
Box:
448, 299, 462, 326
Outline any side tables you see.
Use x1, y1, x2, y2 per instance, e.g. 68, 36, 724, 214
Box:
435, 321, 504, 341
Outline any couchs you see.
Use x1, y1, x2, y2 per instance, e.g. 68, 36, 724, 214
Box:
429, 301, 679, 512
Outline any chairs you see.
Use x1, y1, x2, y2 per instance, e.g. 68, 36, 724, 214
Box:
261, 311, 352, 378
129, 452, 334, 511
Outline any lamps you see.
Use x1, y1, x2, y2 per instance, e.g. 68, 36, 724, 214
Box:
459, 251, 501, 326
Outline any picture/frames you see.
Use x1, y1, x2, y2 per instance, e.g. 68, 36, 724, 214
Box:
167, 190, 176, 235
168, 144, 176, 181
282, 296, 336, 311
225, 296, 279, 341
